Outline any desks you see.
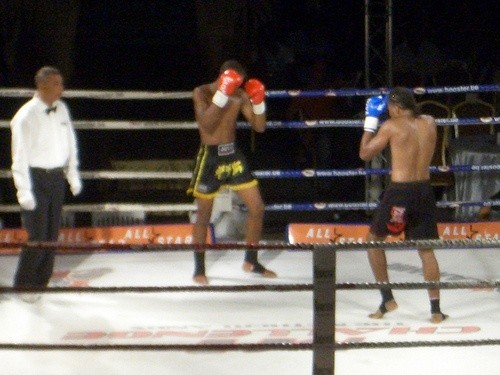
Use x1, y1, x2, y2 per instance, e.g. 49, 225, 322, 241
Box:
447, 134, 500, 218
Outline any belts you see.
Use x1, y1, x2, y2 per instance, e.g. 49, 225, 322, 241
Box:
31, 168, 64, 174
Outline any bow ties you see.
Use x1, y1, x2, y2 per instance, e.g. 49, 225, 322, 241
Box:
46, 106, 56, 113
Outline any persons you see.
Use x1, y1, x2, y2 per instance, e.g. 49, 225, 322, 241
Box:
191, 59, 277, 286
358, 86, 449, 324
9, 66, 83, 289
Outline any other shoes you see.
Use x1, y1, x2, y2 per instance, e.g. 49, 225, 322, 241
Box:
17, 293, 42, 303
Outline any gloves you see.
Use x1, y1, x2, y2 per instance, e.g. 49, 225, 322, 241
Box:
212, 69, 243, 108
245, 79, 266, 115
70, 181, 82, 195
364, 96, 387, 133
16, 190, 36, 211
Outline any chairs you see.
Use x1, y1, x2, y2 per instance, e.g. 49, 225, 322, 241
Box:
415, 100, 496, 200
